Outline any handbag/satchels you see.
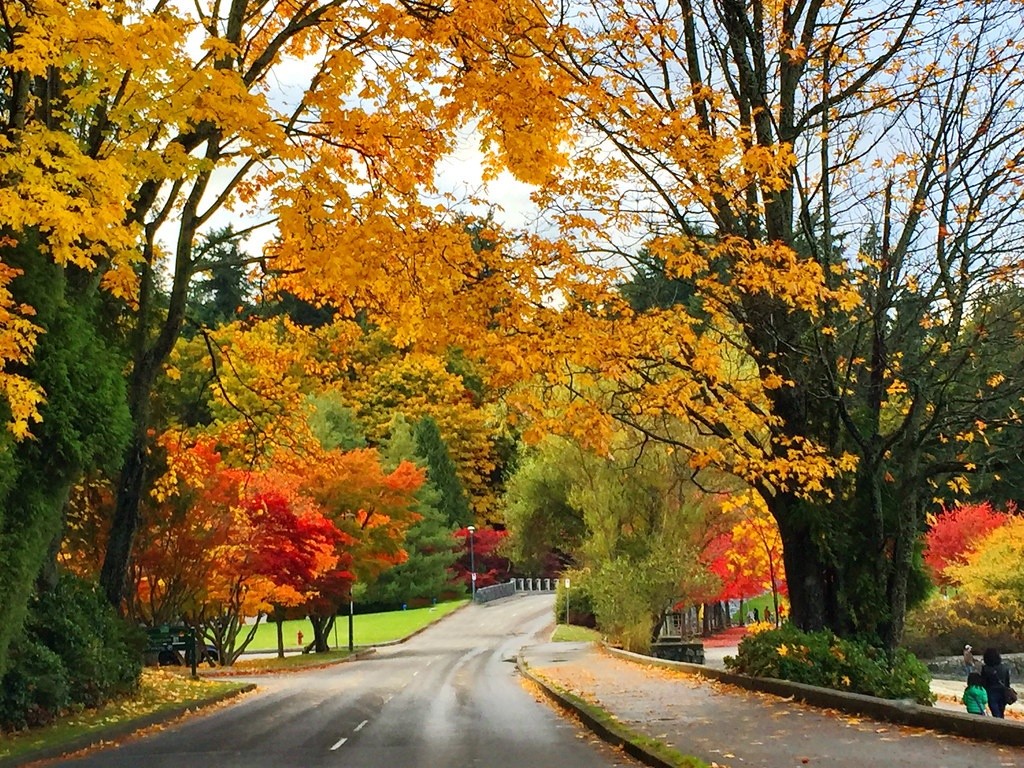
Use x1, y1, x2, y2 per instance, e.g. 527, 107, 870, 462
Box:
982, 710, 989, 717
1003, 686, 1018, 705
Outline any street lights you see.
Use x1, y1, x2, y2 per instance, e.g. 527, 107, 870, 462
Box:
468, 526, 477, 601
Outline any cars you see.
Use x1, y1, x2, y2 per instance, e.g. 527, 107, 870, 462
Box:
145, 626, 219, 666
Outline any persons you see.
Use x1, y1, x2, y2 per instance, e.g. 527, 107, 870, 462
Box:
747, 603, 785, 623
981, 648, 1010, 719
964, 645, 977, 673
961, 672, 989, 715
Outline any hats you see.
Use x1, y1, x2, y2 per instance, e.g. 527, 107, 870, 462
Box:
964, 644, 973, 650
